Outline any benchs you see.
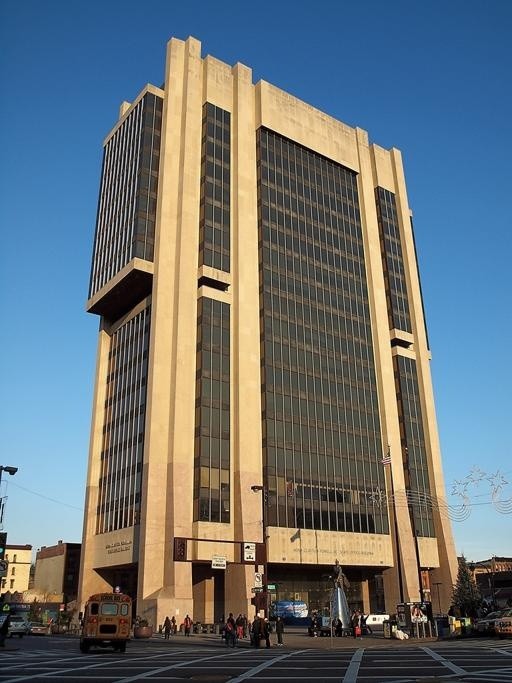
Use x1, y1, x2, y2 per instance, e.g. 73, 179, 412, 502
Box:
308, 627, 367, 638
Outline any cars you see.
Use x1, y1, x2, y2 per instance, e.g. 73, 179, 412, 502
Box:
0, 616, 27, 638
25, 622, 47, 636
473, 610, 512, 639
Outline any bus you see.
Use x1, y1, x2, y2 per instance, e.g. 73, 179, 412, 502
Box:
395, 602, 432, 627
78, 593, 132, 652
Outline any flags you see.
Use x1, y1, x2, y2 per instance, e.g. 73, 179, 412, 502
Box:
379, 450, 391, 465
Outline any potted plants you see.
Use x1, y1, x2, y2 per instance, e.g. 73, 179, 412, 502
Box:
134, 619, 152, 638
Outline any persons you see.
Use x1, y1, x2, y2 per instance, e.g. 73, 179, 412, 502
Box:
449, 600, 495, 619
0, 614, 12, 647
222, 613, 285, 648
310, 608, 374, 637
332, 559, 344, 589
162, 614, 192, 640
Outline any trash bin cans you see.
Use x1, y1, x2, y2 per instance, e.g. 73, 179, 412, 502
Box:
383, 620, 397, 639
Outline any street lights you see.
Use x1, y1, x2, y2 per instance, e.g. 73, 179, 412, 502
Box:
0, 465, 18, 491
251, 485, 270, 621
